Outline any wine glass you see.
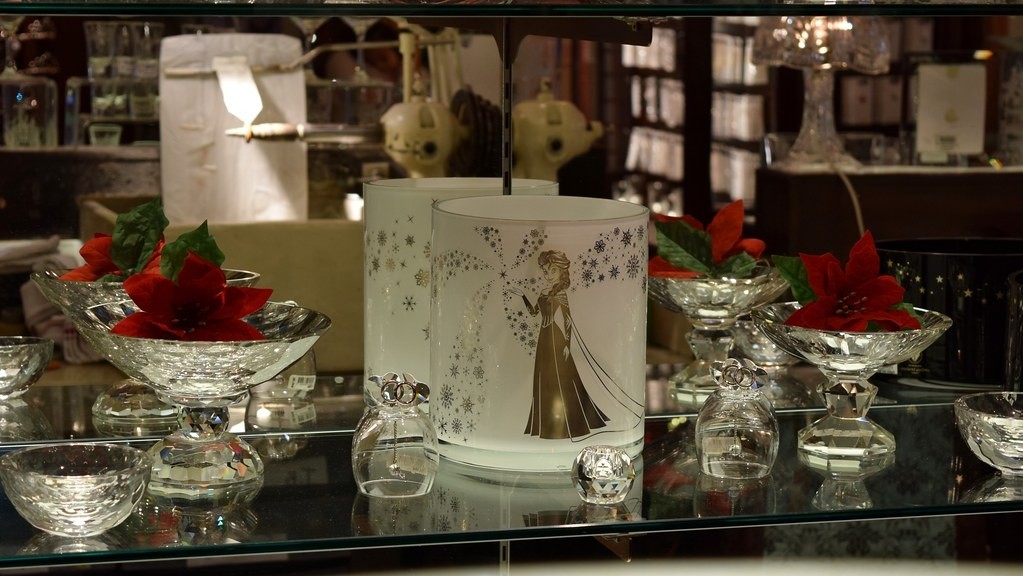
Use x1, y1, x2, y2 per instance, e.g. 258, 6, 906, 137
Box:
29, 266, 261, 423
291, 16, 333, 88
339, 15, 385, 85
68, 294, 333, 487
750, 299, 951, 455
646, 262, 790, 392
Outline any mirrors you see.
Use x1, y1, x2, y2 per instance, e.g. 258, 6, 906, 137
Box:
3, 14, 1021, 448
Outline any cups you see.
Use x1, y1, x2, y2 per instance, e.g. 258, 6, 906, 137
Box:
65, 18, 236, 147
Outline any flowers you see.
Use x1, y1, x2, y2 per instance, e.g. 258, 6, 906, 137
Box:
783, 232, 923, 340
58, 194, 168, 284
645, 199, 764, 277
109, 219, 274, 344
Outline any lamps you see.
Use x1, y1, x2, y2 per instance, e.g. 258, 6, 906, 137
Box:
751, 0, 889, 171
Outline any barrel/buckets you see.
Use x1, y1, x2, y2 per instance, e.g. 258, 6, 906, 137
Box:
368, 439, 434, 533
361, 177, 560, 414
428, 193, 650, 469
430, 436, 643, 534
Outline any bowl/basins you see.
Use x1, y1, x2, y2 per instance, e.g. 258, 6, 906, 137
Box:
954, 390, 1022, 476
0, 335, 56, 402
0, 444, 152, 537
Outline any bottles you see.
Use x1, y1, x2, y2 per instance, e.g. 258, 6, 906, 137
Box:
694, 357, 780, 479
352, 372, 439, 498
249, 348, 316, 398
